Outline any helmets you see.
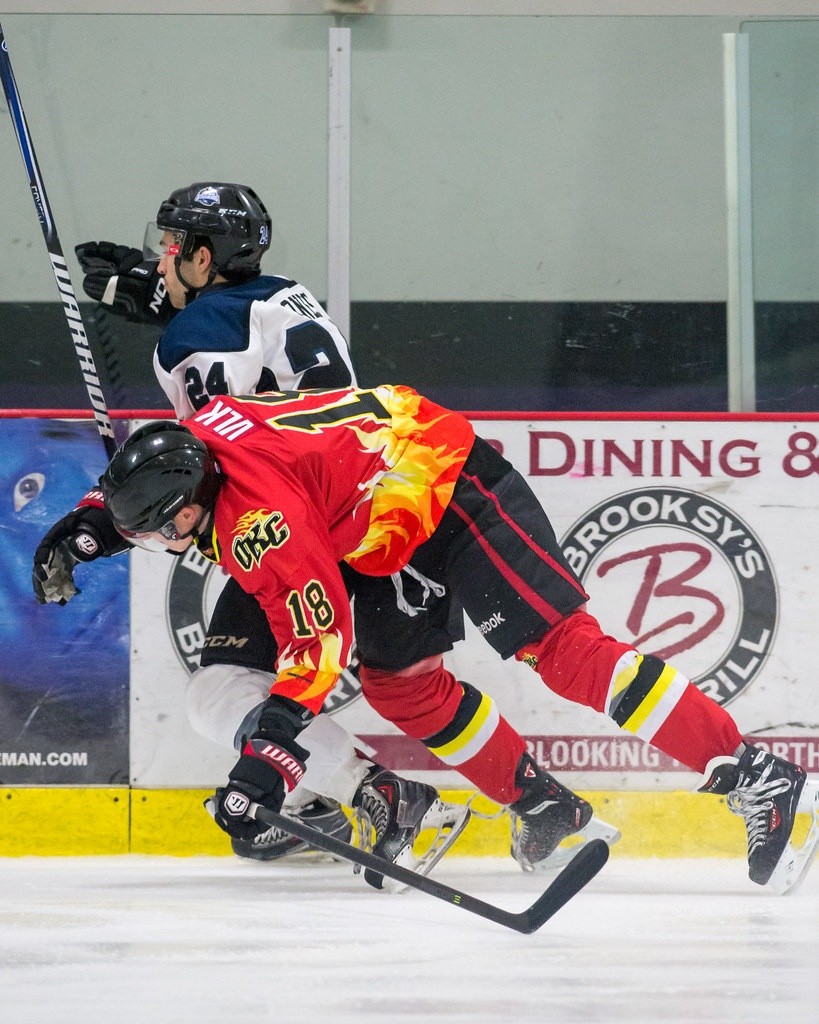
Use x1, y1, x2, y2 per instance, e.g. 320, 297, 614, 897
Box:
101, 421, 224, 534
156, 182, 272, 284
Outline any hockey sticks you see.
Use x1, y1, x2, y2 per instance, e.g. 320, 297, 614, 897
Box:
0, 23, 121, 462
215, 787, 610, 936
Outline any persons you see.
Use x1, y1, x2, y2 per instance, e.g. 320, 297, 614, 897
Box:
33, 181, 819, 893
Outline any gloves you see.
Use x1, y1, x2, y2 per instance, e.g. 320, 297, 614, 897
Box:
75, 241, 182, 325
203, 730, 310, 841
32, 485, 139, 607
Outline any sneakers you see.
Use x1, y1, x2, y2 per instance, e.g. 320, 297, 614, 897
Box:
509, 752, 621, 872
346, 760, 471, 894
231, 796, 354, 869
691, 740, 819, 896
